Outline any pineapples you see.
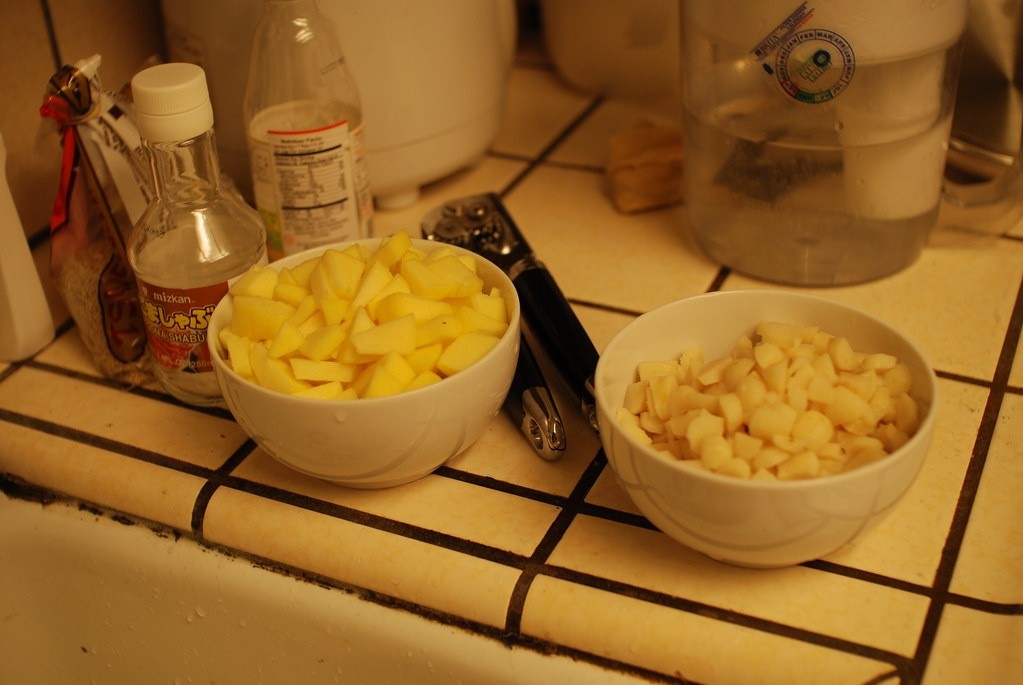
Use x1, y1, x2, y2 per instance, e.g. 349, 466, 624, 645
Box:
218, 231, 508, 403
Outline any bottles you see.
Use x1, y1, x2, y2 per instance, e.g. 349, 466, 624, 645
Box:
118, 58, 274, 405
242, 0, 375, 261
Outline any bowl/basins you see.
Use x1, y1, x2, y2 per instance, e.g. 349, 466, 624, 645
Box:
599, 288, 938, 568
204, 234, 522, 488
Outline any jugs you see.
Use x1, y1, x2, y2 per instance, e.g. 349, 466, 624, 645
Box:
673, 0, 1021, 288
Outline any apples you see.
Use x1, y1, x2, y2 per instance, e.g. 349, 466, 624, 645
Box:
600, 322, 919, 480
